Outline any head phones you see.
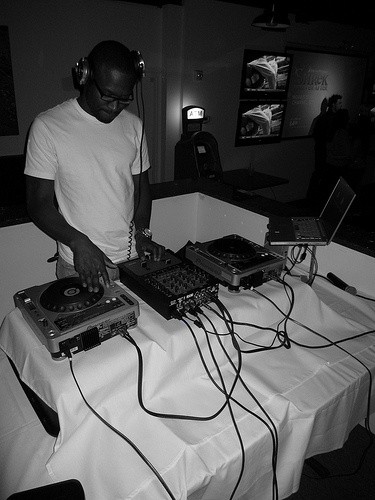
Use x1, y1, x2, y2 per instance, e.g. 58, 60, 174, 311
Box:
73, 48, 146, 88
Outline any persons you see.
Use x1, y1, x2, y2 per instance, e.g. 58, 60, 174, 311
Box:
305, 93, 349, 196
23, 39, 167, 292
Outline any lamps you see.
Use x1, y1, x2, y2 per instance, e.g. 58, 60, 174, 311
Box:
252, 0, 291, 29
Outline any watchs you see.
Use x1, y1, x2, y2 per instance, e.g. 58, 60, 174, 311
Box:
134, 228, 153, 240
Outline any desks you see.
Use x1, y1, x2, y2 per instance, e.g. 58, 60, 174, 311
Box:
0, 259, 375, 500
218, 169, 289, 201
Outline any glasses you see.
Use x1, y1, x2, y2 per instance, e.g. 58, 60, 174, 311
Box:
94, 80, 135, 104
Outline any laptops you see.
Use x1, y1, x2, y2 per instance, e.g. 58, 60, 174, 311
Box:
268, 175, 357, 245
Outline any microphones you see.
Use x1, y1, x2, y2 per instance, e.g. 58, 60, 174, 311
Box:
327, 272, 358, 296
